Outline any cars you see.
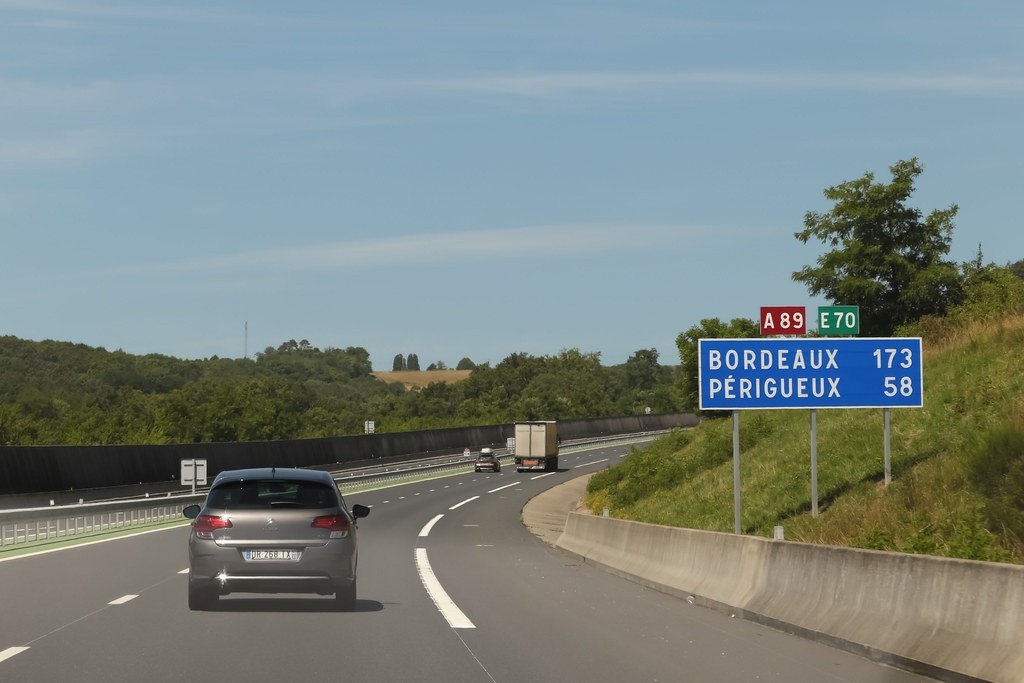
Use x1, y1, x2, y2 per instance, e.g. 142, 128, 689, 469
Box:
474, 447, 501, 474
183, 469, 372, 612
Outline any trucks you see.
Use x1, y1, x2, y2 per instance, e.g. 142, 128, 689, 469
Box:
514, 420, 562, 473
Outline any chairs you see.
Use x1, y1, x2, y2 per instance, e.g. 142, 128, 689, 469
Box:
237, 484, 259, 503
296, 485, 321, 503
